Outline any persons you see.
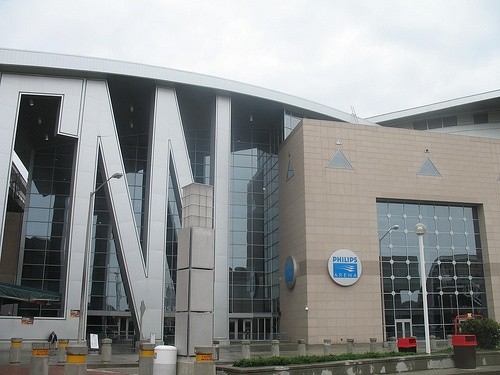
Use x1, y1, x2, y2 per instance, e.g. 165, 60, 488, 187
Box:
48, 330, 58, 350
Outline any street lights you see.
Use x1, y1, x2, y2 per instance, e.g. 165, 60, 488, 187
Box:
78, 172, 124, 345
379, 225, 400, 342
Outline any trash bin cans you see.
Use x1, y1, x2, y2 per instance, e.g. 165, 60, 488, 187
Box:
452, 335, 478, 369
397, 337, 417, 352
